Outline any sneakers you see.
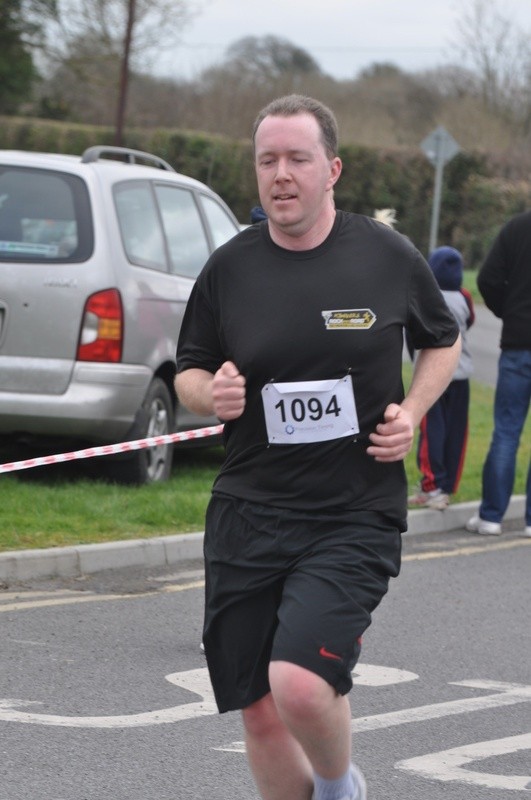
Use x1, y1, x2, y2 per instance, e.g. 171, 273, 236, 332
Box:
407, 487, 449, 509
466, 515, 502, 534
524, 526, 531, 537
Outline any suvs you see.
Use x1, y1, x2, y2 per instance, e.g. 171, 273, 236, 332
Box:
0, 140, 246, 490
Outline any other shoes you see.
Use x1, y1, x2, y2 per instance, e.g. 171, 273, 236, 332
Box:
309, 763, 367, 800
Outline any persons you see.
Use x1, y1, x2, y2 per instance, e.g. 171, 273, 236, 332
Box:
406, 244, 476, 512
465, 184, 531, 538
173, 95, 463, 800
249, 205, 267, 224
352, 203, 398, 229
